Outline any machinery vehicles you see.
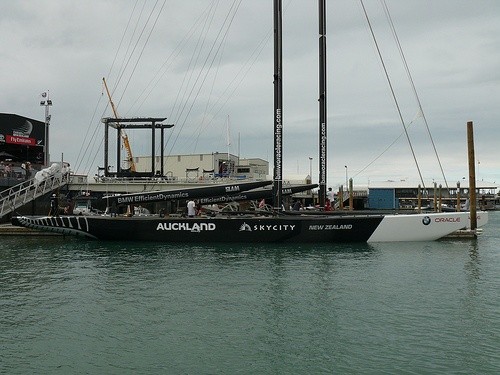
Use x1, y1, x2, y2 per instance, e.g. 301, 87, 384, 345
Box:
100, 77, 135, 172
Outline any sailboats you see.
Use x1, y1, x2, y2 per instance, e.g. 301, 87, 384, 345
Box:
8, 2, 489, 245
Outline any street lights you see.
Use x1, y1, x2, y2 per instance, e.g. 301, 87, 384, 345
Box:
345, 165, 348, 191
40, 89, 53, 166
308, 157, 313, 185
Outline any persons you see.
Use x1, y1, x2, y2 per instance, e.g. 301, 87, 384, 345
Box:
328, 188, 336, 206
21, 160, 27, 178
47, 194, 56, 217
293, 200, 305, 211
324, 203, 332, 211
26, 161, 32, 179
64, 204, 73, 216
195, 199, 201, 217
5, 164, 10, 175
187, 197, 198, 218
